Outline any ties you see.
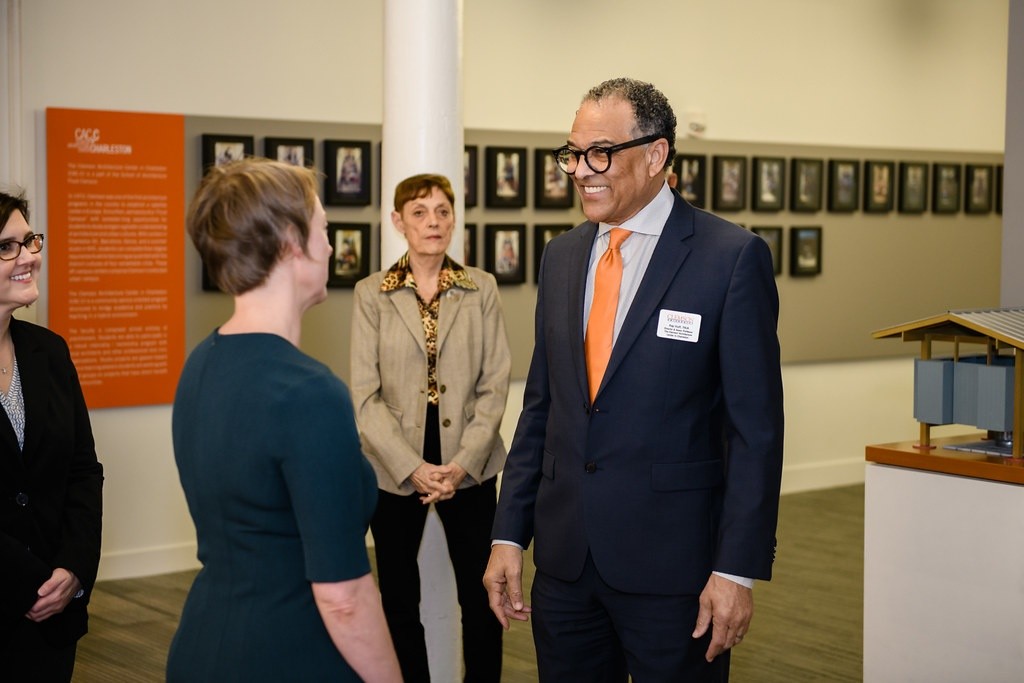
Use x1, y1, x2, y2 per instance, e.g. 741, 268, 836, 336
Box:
584, 228, 633, 407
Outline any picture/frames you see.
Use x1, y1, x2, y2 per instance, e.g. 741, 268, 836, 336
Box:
485, 223, 528, 285
464, 224, 477, 267
485, 147, 527, 209
202, 133, 315, 291
326, 221, 371, 287
464, 146, 478, 208
322, 140, 372, 206
534, 148, 574, 209
667, 153, 1004, 277
533, 223, 574, 284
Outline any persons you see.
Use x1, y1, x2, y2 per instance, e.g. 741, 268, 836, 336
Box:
348, 175, 513, 683
0, 194, 103, 683
479, 78, 788, 683
167, 161, 407, 683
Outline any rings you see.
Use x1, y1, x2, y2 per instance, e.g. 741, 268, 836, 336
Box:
736, 634, 743, 640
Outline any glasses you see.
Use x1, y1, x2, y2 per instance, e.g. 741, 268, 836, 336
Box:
553, 133, 672, 175
0, 233, 44, 261
